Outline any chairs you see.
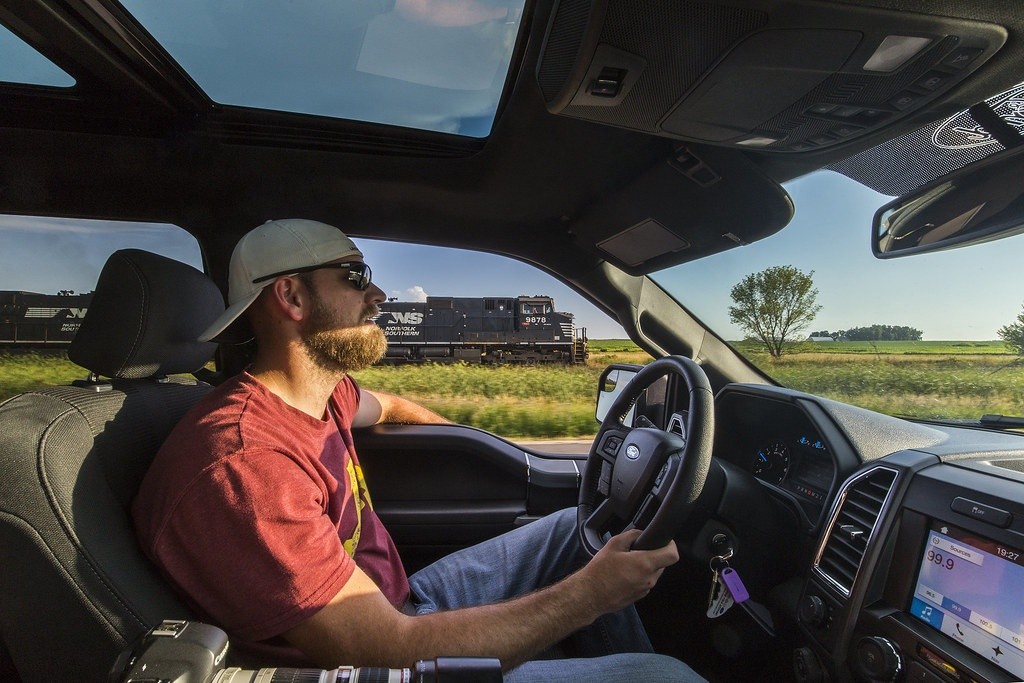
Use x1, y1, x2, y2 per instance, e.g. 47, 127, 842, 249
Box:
0, 249, 228, 683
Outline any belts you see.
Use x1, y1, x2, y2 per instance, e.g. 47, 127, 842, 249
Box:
400, 585, 422, 616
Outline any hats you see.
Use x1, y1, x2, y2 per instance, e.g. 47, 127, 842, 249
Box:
197, 218, 363, 345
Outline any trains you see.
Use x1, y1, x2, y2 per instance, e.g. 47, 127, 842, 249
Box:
0, 291, 589, 365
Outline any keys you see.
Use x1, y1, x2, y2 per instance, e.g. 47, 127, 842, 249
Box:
714, 537, 734, 561
709, 570, 718, 607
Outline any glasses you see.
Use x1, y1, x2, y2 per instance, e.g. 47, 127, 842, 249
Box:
253, 261, 371, 291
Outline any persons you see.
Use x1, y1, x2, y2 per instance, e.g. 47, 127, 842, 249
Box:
131, 218, 710, 683
